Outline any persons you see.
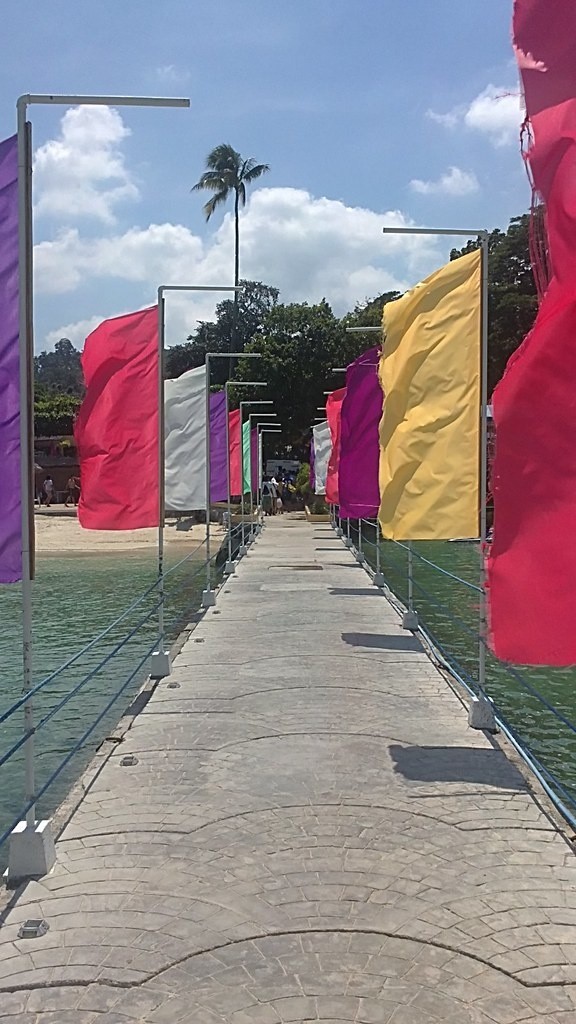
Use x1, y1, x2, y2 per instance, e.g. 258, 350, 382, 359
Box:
262, 476, 304, 515
64, 474, 79, 508
43, 474, 53, 507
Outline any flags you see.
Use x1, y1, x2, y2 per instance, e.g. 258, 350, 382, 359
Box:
313, 419, 334, 495
485, 0, 576, 667
259, 433, 262, 489
228, 409, 242, 496
250, 427, 257, 491
242, 419, 251, 495
338, 341, 384, 518
377, 249, 481, 540
309, 436, 316, 489
209, 390, 228, 504
163, 364, 207, 509
74, 301, 159, 531
0, 122, 41, 584
326, 385, 348, 504
